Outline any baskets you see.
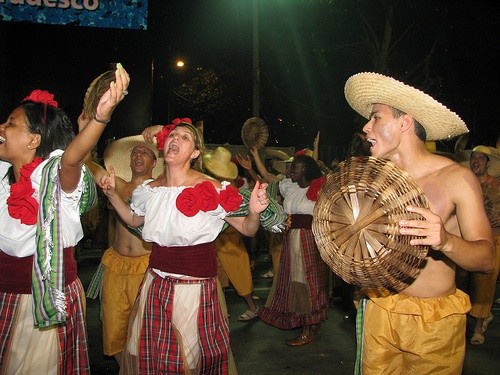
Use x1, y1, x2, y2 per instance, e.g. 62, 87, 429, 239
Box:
310, 154, 431, 297
83, 70, 116, 120
241, 118, 269, 150
480, 183, 500, 220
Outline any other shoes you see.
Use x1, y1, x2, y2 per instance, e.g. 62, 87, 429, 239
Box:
286, 332, 315, 346
260, 271, 275, 278
470, 333, 485, 344
482, 312, 493, 332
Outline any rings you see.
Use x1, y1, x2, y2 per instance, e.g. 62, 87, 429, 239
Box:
121, 90, 128, 95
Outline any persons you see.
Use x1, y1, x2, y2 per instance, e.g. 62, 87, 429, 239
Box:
344, 72, 497, 375
0, 64, 500, 375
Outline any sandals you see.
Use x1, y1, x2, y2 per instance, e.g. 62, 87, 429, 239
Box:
237, 305, 260, 320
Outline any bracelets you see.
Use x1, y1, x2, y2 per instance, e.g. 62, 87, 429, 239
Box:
93, 115, 111, 124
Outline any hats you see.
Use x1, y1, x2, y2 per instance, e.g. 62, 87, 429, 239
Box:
266, 149, 289, 160
202, 147, 238, 180
455, 145, 500, 178
344, 72, 471, 142
102, 136, 166, 182
272, 157, 295, 175
